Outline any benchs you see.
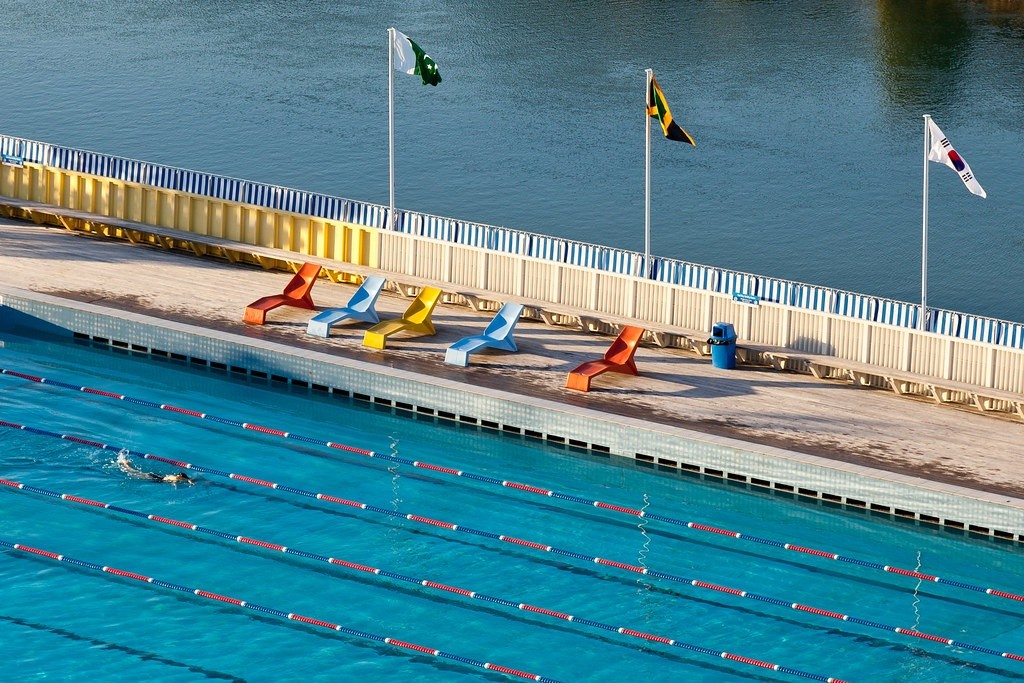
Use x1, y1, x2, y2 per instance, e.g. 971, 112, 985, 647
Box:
0, 194, 1024, 423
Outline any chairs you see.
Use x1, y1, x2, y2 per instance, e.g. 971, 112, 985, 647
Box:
564, 325, 645, 392
444, 302, 525, 368
243, 262, 443, 350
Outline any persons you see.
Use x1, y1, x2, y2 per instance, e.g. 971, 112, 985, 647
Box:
123, 460, 195, 487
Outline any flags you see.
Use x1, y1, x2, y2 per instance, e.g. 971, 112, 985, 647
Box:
645, 69, 696, 146
392, 27, 442, 86
926, 117, 987, 198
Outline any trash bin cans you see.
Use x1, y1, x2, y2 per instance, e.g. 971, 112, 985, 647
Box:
710, 321, 738, 369
